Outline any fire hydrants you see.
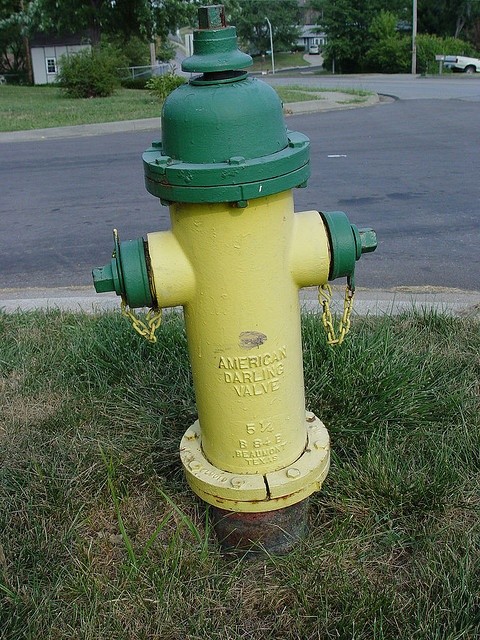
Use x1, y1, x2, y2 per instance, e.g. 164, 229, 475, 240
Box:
91, 4, 379, 563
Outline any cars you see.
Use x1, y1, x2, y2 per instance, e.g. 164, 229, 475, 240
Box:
309, 44, 320, 56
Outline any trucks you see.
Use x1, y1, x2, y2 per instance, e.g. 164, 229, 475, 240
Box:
444, 55, 480, 74
